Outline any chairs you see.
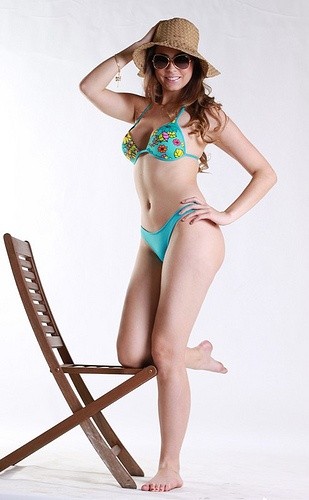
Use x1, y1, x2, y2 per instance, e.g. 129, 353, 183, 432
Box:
0, 233, 158, 490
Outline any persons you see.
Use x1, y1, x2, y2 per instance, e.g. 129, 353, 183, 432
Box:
80, 17, 278, 492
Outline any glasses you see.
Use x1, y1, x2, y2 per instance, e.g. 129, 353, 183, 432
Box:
149, 54, 193, 71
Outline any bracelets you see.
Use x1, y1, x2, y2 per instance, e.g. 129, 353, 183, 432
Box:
113, 55, 121, 82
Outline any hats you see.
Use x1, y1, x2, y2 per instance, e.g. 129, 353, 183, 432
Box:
130, 18, 221, 78
